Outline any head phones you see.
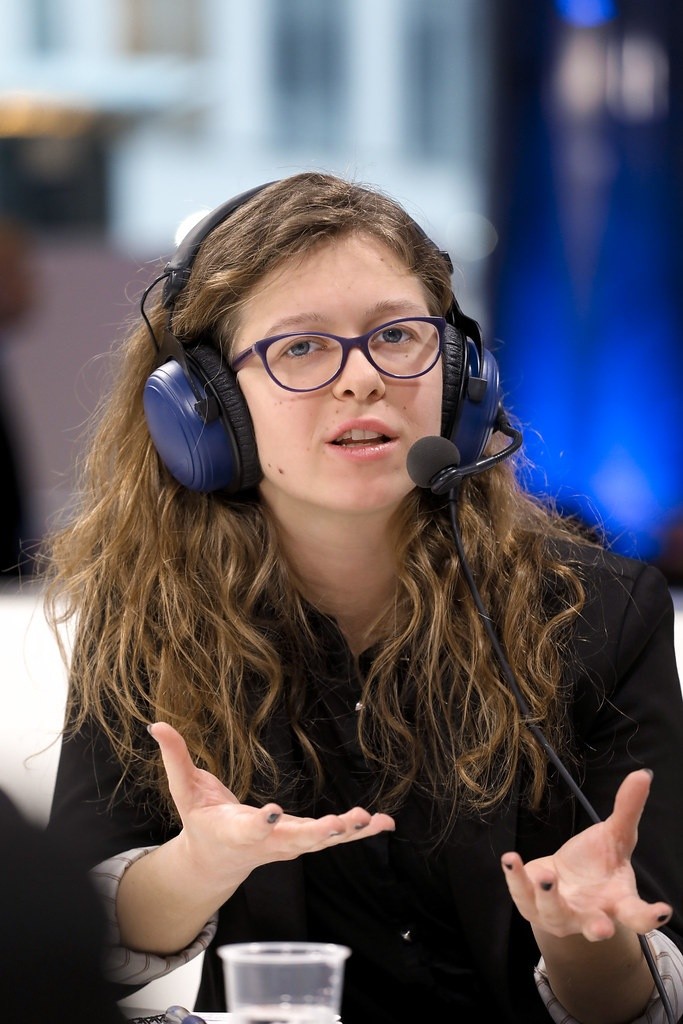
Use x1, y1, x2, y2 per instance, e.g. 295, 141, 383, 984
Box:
140, 179, 502, 493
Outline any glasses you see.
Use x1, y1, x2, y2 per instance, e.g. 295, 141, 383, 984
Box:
227, 316, 448, 393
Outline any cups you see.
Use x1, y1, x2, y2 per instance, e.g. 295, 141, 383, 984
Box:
217, 942, 352, 1024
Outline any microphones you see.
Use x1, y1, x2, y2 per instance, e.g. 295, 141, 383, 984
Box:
406, 412, 523, 493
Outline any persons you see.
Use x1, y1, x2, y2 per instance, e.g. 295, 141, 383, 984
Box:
41, 174, 683, 1024
